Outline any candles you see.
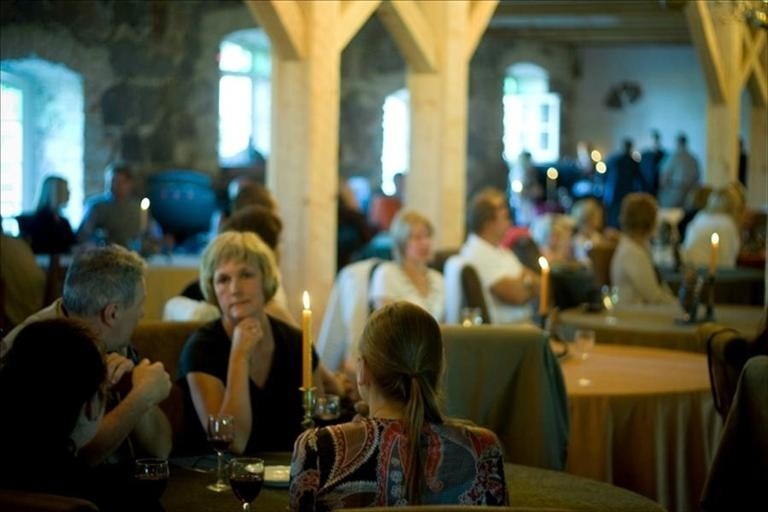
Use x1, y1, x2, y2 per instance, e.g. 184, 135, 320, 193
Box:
708, 230, 719, 279
140, 196, 150, 234
299, 286, 315, 391
536, 253, 551, 315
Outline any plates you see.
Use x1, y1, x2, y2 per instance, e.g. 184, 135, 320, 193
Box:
264, 466, 291, 487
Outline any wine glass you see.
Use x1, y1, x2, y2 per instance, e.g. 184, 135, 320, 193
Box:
313, 393, 341, 427
227, 456, 264, 512
572, 330, 596, 366
208, 412, 235, 493
133, 459, 168, 512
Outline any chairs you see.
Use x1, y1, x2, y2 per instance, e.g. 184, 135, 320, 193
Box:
694, 320, 752, 425
442, 252, 494, 324
586, 246, 618, 295
336, 255, 394, 372
437, 322, 573, 473
698, 355, 768, 512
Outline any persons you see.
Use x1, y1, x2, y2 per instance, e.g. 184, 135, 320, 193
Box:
74, 167, 163, 252
575, 141, 594, 177
24, 177, 75, 256
338, 177, 380, 269
508, 151, 542, 225
0, 216, 46, 328
0, 319, 107, 509
370, 209, 444, 324
460, 185, 541, 327
677, 187, 712, 241
209, 176, 246, 237
571, 198, 604, 266
603, 136, 649, 227
527, 212, 600, 308
178, 201, 294, 324
177, 230, 345, 459
289, 300, 510, 511
659, 133, 700, 209
390, 174, 402, 199
607, 191, 674, 305
684, 187, 742, 271
3, 243, 173, 462
640, 129, 668, 190
